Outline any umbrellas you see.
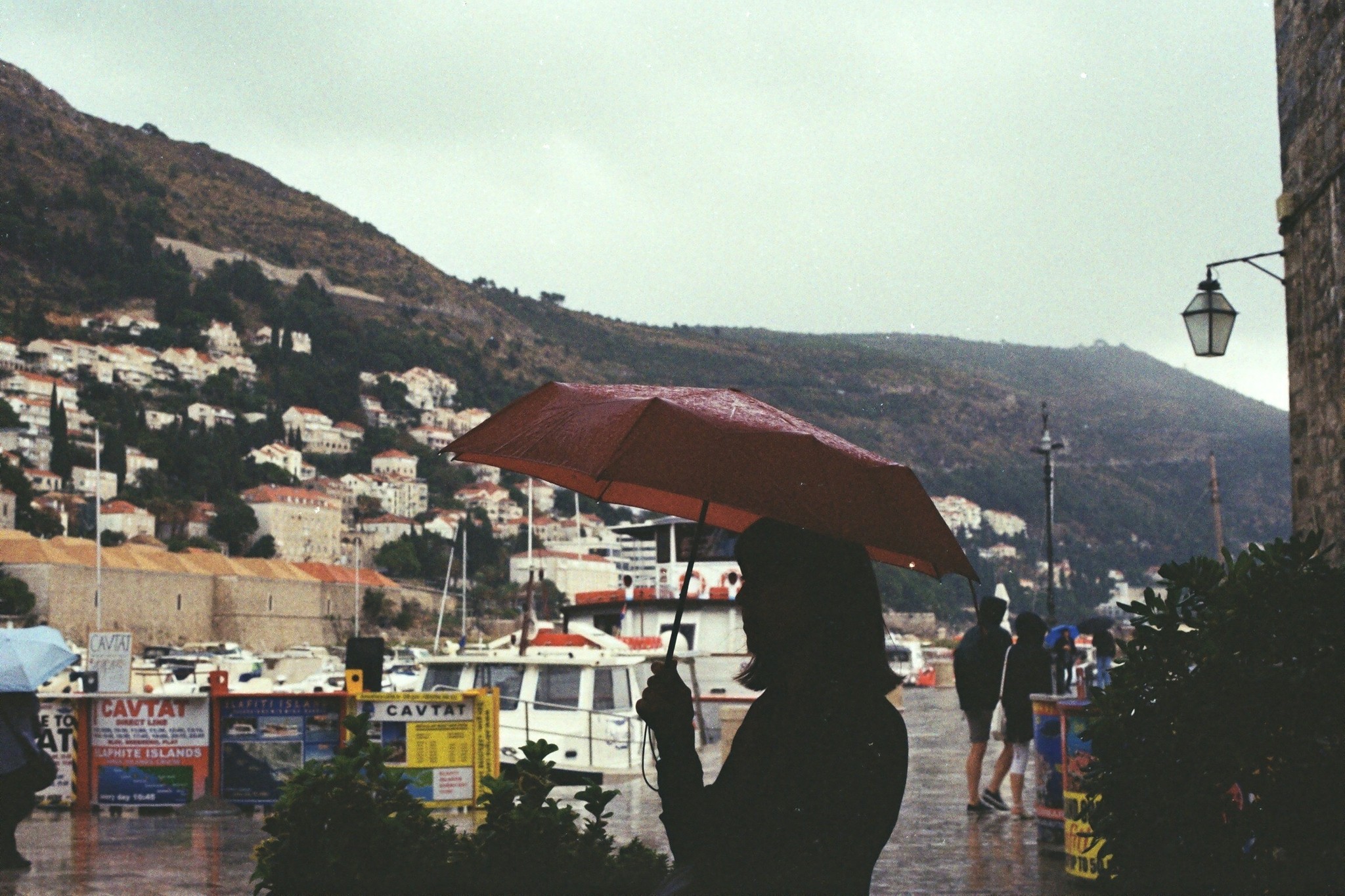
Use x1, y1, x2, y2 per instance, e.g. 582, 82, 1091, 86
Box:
0, 627, 75, 693
438, 378, 983, 792
1045, 624, 1080, 648
1076, 614, 1115, 633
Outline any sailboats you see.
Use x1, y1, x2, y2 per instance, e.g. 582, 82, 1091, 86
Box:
55, 430, 759, 783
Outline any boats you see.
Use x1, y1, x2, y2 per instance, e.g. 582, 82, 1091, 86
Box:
885, 626, 926, 678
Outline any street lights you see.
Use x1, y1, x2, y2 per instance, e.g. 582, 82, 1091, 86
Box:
1027, 399, 1064, 628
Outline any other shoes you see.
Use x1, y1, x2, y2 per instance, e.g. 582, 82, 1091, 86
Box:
1010, 804, 1034, 821
981, 789, 1011, 811
968, 801, 991, 815
1065, 686, 1072, 694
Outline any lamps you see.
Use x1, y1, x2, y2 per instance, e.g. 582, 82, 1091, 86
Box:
1180, 248, 1286, 357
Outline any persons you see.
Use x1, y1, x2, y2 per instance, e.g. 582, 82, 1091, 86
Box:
950, 597, 1119, 819
636, 517, 909, 896
0, 689, 61, 867
